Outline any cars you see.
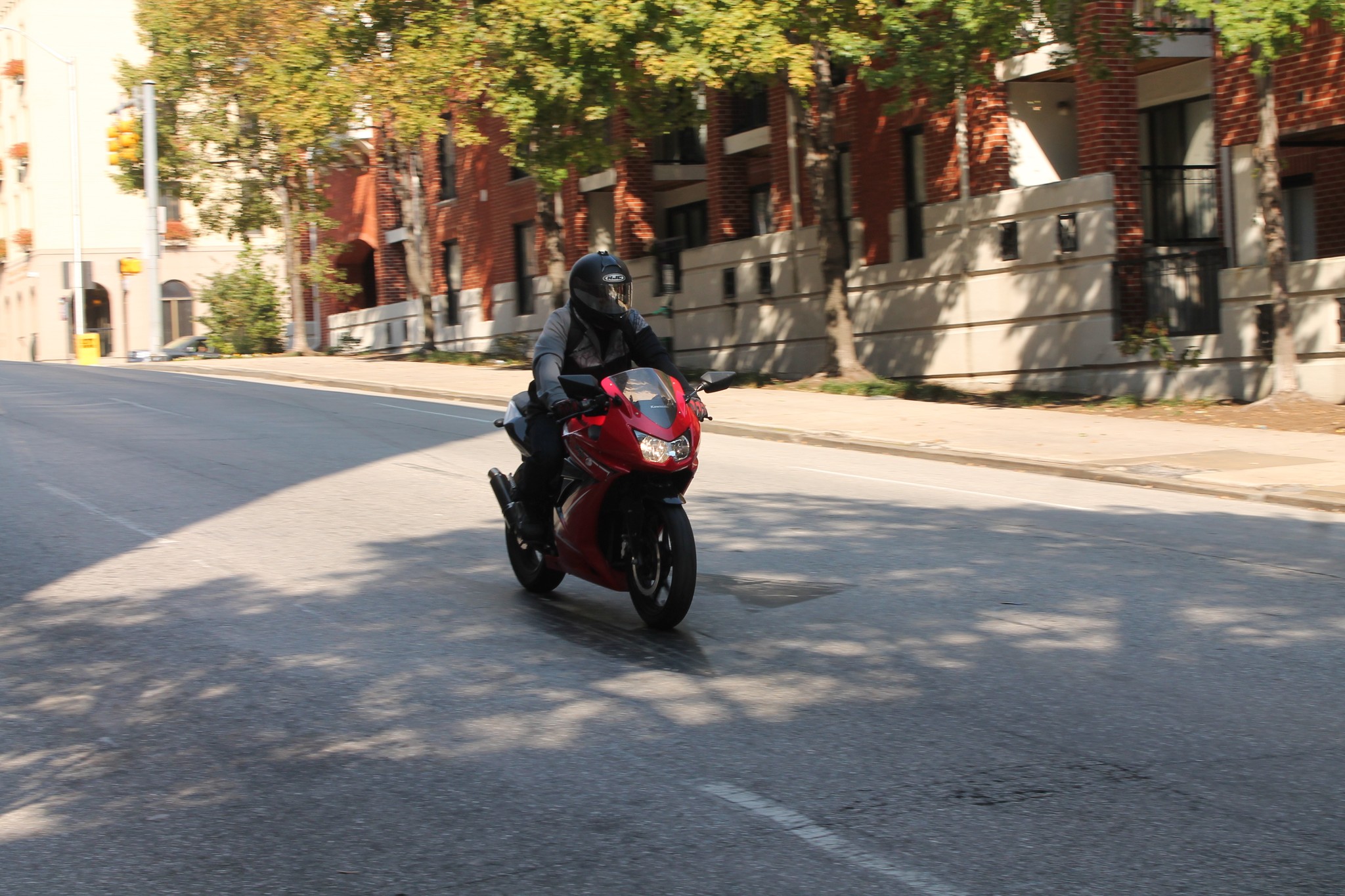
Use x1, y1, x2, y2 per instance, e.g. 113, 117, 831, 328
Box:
128, 335, 219, 362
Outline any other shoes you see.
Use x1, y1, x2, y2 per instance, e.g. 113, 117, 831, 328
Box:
512, 501, 545, 540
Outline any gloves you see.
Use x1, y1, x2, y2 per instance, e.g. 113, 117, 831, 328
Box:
687, 397, 707, 422
551, 399, 582, 420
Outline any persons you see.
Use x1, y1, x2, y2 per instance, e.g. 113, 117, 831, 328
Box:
517, 251, 708, 544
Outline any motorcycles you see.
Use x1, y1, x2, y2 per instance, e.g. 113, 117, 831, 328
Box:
487, 366, 737, 632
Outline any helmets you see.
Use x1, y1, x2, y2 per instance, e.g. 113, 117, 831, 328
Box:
569, 251, 633, 330
